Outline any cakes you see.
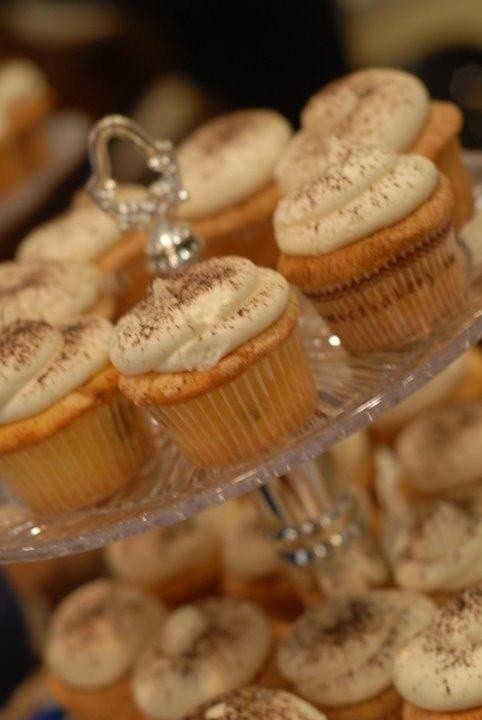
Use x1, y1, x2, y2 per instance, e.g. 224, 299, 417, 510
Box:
298, 63, 474, 232
319, 346, 482, 505
273, 132, 467, 353
155, 108, 295, 272
109, 258, 331, 470
0, 316, 160, 515
1, 256, 117, 336
14, 183, 155, 324
1, 57, 57, 206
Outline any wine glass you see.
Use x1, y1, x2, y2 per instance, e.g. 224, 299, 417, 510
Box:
5, 154, 479, 614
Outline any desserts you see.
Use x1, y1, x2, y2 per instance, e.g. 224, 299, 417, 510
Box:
44, 503, 482, 720
